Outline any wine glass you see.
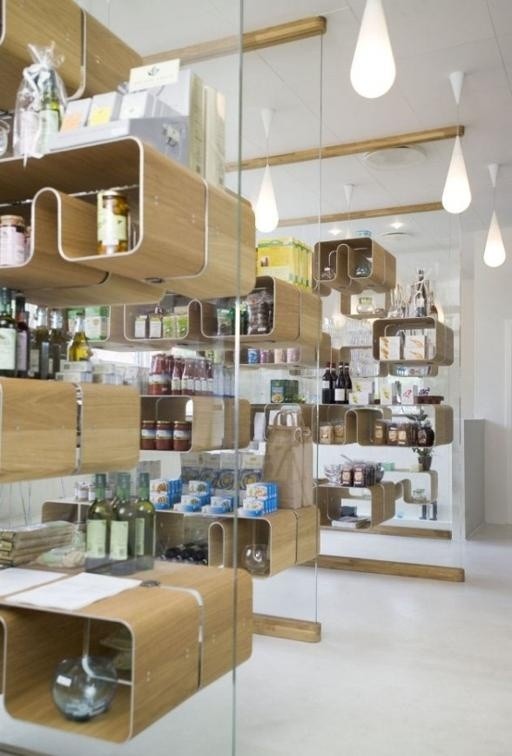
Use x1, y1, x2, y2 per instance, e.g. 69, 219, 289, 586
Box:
323, 464, 341, 487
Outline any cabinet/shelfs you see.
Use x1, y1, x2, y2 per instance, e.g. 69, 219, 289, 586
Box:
4, 4, 202, 743
34, 229, 465, 584
82, 6, 255, 684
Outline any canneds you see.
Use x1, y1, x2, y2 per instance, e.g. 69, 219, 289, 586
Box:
141, 421, 191, 451
0, 215, 25, 266
97, 191, 131, 253
25, 226, 31, 261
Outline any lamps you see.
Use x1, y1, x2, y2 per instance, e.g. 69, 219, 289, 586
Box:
481, 159, 508, 268
254, 104, 283, 232
347, 1, 397, 99
440, 65, 475, 214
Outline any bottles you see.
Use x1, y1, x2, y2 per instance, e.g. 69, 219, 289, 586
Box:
1, 284, 91, 380
321, 361, 352, 402
415, 269, 427, 317
356, 296, 375, 314
407, 286, 415, 316
427, 291, 438, 321
39, 71, 63, 151
372, 419, 432, 446
148, 353, 231, 396
147, 307, 163, 339
20, 73, 39, 154
87, 472, 150, 564
154, 538, 209, 567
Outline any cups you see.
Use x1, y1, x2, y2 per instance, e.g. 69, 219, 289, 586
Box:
322, 315, 371, 347
247, 348, 297, 363
49, 657, 118, 721
241, 543, 271, 577
